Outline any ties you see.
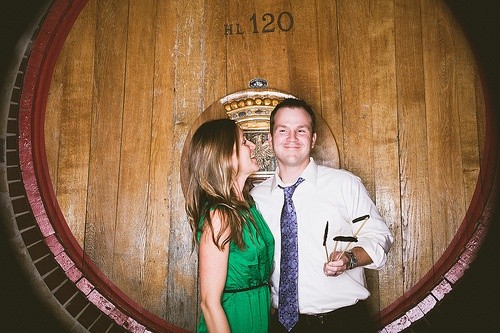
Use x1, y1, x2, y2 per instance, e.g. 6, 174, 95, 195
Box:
277, 177, 305, 332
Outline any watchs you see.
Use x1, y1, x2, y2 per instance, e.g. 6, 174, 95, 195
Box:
344, 251, 358, 269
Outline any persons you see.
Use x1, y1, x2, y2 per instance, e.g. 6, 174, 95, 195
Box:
248, 98, 395, 333
185, 118, 275, 333
